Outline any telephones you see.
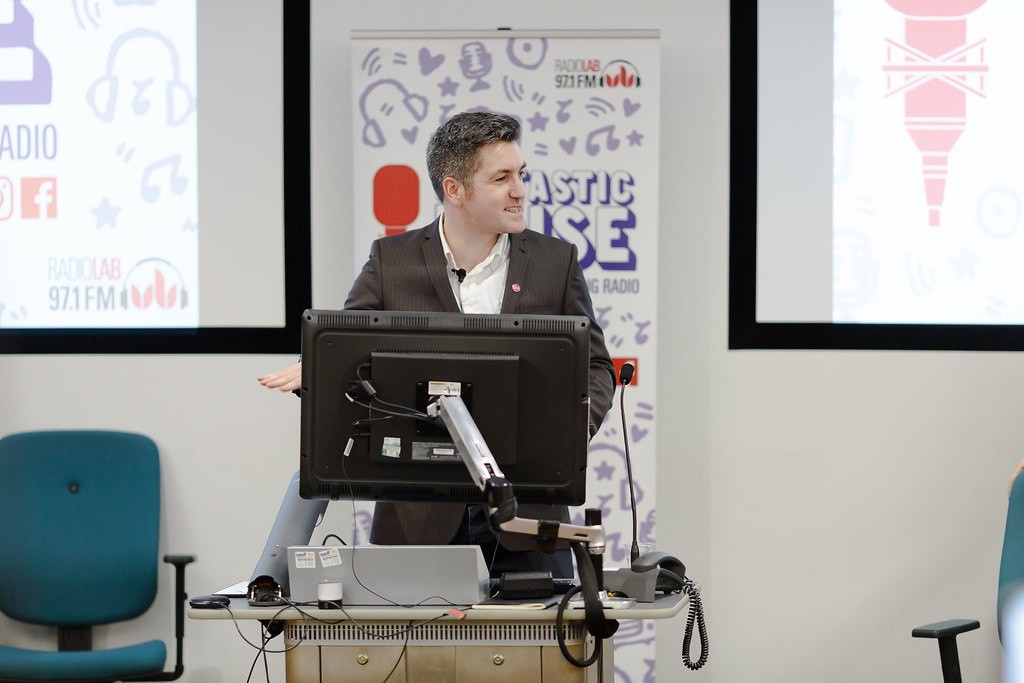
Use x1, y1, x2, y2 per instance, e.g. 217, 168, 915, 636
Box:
602, 552, 686, 602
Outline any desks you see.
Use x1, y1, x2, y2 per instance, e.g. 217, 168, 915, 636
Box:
187, 583, 701, 683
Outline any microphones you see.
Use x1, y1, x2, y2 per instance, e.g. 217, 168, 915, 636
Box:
620, 364, 639, 562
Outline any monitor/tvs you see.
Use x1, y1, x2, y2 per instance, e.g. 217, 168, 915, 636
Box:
298, 311, 591, 507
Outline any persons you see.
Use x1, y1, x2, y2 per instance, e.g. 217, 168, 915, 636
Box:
258, 111, 616, 601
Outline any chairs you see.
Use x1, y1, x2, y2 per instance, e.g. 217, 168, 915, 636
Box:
0, 430, 195, 683
